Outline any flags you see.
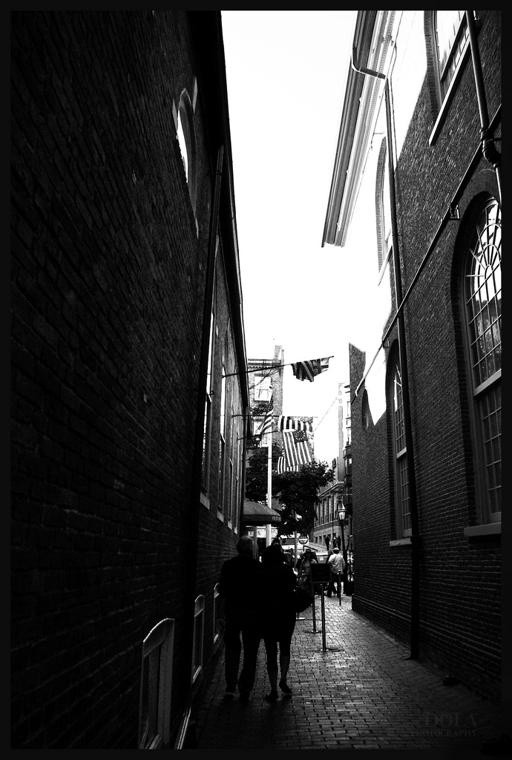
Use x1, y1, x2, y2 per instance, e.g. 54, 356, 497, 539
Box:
291, 358, 329, 382
282, 431, 312, 467
277, 415, 314, 432
278, 457, 299, 474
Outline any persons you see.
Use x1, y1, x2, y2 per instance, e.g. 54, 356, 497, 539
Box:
262, 544, 296, 694
222, 537, 262, 701
327, 547, 346, 598
